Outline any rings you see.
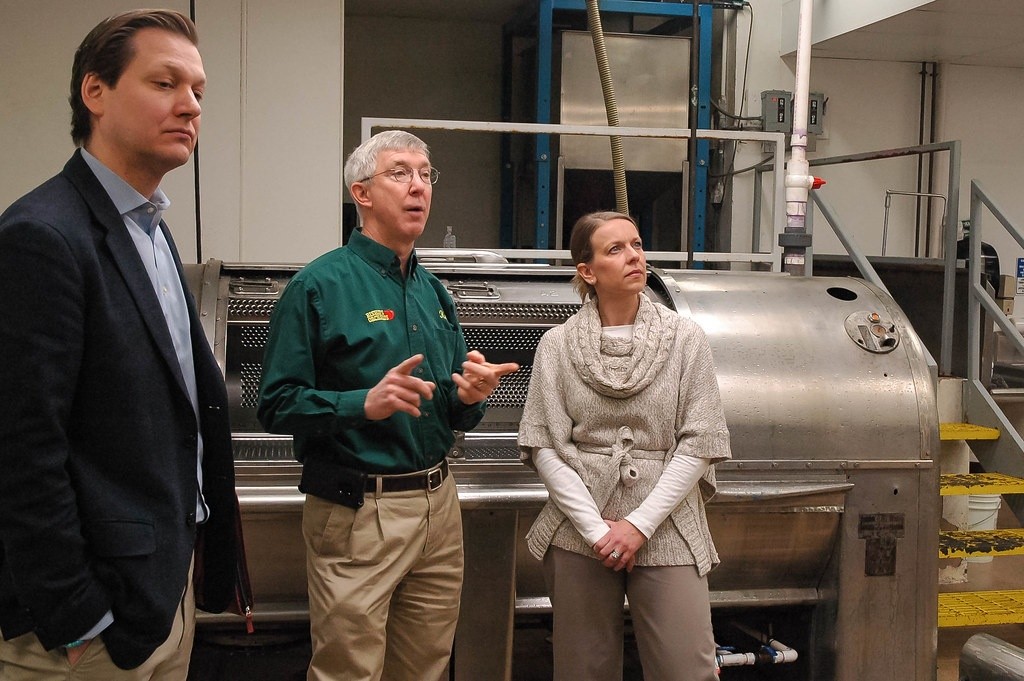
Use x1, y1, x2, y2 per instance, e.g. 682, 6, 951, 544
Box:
478, 377, 484, 389
612, 552, 619, 559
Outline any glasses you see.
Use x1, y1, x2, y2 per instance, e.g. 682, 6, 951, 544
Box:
358, 165, 440, 185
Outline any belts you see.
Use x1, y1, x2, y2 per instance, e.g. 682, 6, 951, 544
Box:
366, 459, 448, 492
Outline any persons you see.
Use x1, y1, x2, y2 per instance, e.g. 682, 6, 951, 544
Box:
0, 9, 254, 681
517, 211, 732, 681
255, 130, 519, 681
